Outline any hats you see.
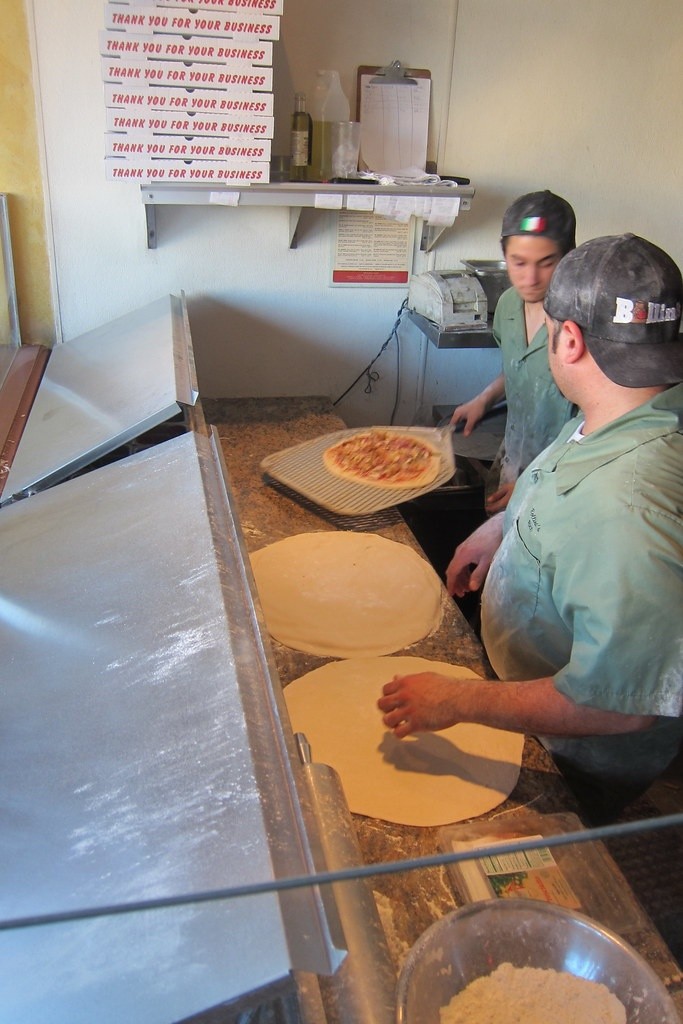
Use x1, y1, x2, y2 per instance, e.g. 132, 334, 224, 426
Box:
545, 233, 683, 388
503, 189, 577, 257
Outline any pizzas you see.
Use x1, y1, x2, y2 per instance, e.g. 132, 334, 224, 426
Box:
323, 431, 440, 488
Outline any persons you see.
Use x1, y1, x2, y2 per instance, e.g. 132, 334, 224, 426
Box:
447, 190, 584, 520
377, 231, 683, 828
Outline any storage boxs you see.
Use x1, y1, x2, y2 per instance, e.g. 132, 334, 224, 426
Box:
97, 0, 283, 182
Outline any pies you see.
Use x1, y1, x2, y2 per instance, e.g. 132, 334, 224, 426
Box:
281, 656, 526, 827
250, 529, 444, 658
